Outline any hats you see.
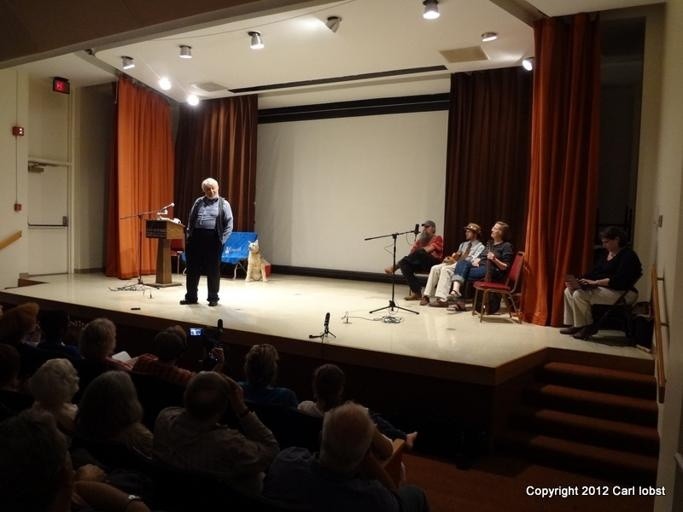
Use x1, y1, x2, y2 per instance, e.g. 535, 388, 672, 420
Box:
422, 220, 435, 227
463, 222, 482, 235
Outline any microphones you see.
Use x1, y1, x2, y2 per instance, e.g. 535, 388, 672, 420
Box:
159, 202, 176, 211
414, 224, 420, 241
323, 312, 331, 326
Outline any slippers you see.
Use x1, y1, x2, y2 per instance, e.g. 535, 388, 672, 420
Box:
446, 290, 461, 297
448, 304, 464, 311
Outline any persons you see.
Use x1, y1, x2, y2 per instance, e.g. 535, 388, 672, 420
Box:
557, 224, 643, 340
420, 223, 485, 307
180, 174, 234, 308
0, 298, 429, 511
446, 220, 513, 313
383, 219, 445, 302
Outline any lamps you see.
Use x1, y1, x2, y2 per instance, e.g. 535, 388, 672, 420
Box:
121, 55, 135, 70
180, 44, 191, 59
248, 30, 264, 49
421, 0, 440, 19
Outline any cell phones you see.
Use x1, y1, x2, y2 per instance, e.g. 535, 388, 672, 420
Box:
188, 327, 206, 337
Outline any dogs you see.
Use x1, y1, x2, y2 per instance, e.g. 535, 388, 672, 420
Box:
245, 239, 267, 283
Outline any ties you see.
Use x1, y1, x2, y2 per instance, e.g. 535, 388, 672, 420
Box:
460, 242, 472, 261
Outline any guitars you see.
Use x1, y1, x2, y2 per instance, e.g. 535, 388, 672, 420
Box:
475, 240, 502, 313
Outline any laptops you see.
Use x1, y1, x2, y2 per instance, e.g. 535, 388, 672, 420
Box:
563, 273, 598, 289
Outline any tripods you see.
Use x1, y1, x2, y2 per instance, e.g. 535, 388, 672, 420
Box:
117, 208, 164, 291
320, 326, 337, 338
364, 230, 421, 315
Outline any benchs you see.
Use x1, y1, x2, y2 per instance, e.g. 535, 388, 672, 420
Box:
181, 229, 256, 279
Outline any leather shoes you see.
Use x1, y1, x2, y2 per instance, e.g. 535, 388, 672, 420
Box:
429, 299, 447, 307
210, 302, 217, 306
420, 297, 427, 305
405, 292, 420, 300
574, 325, 598, 339
560, 327, 581, 334
385, 266, 394, 274
180, 300, 197, 304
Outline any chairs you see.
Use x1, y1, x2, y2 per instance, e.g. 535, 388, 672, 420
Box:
584, 273, 643, 343
169, 240, 183, 274
471, 250, 525, 324
